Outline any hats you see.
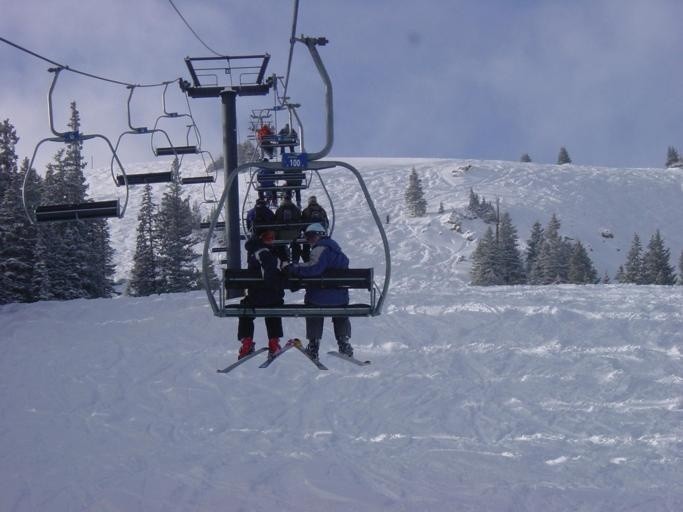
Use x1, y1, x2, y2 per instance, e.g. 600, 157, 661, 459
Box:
305, 223, 325, 233
308, 196, 316, 205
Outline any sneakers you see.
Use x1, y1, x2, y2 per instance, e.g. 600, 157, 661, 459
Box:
305, 341, 320, 360
337, 338, 353, 355
267, 338, 281, 360
237, 338, 255, 360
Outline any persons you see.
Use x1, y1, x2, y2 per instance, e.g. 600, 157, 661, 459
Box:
237, 229, 285, 356
245, 199, 275, 259
256, 123, 273, 160
257, 158, 279, 207
281, 224, 357, 354
284, 170, 306, 209
277, 123, 297, 155
302, 196, 329, 263
275, 194, 302, 264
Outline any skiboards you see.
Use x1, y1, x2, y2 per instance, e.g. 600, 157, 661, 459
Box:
293, 338, 371, 370
216, 339, 296, 373
